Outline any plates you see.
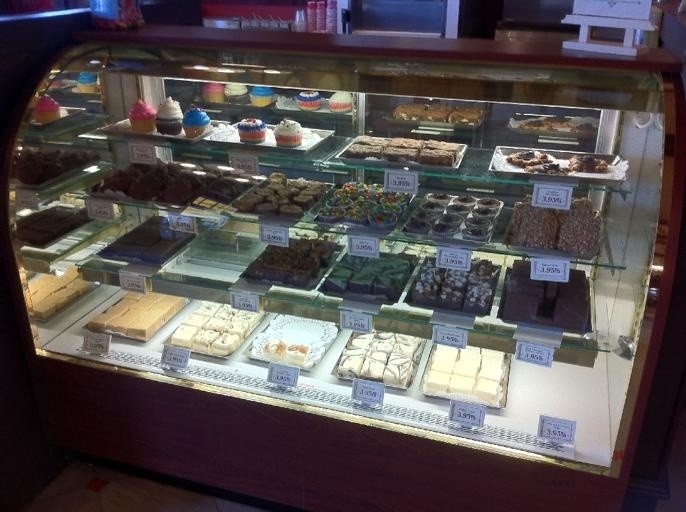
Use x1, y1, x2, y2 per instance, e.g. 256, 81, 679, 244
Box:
267, 97, 353, 120
23, 105, 87, 132
29, 280, 101, 324
239, 237, 345, 291
86, 182, 190, 213
97, 117, 231, 145
488, 145, 627, 186
381, 108, 492, 130
402, 256, 502, 317
201, 118, 337, 156
313, 183, 416, 237
243, 313, 343, 376
330, 328, 427, 391
223, 177, 336, 226
8, 156, 103, 191
334, 134, 467, 173
416, 341, 513, 409
192, 93, 279, 112
507, 115, 596, 139
15, 205, 96, 250
82, 291, 194, 344
96, 216, 198, 269
314, 250, 422, 306
400, 191, 504, 248
502, 202, 598, 259
162, 300, 270, 361
495, 267, 593, 335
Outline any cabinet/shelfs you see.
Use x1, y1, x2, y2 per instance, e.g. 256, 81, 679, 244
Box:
3, 22, 685, 512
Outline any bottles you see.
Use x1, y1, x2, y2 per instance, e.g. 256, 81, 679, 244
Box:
252, 18, 259, 28
271, 20, 277, 29
234, 18, 240, 29
308, 1, 317, 30
280, 19, 287, 30
296, 10, 305, 30
241, 19, 249, 27
262, 20, 267, 27
326, 0, 336, 32
317, 2, 325, 29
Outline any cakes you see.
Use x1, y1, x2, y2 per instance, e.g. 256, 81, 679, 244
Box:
520, 116, 597, 138
225, 85, 248, 103
337, 330, 426, 391
420, 201, 445, 218
212, 333, 242, 356
280, 204, 304, 214
334, 182, 414, 215
192, 330, 219, 354
438, 214, 463, 228
286, 345, 308, 367
236, 232, 258, 248
448, 206, 468, 217
18, 228, 53, 248
324, 254, 415, 303
553, 296, 589, 331
76, 73, 98, 95
325, 197, 352, 211
265, 340, 284, 360
507, 202, 558, 250
292, 195, 314, 208
50, 81, 64, 89
21, 210, 48, 226
520, 195, 593, 216
476, 378, 501, 404
129, 100, 156, 132
559, 215, 600, 260
204, 304, 259, 339
570, 155, 608, 173
288, 178, 327, 200
422, 372, 451, 395
508, 151, 555, 167
449, 374, 477, 399
297, 91, 323, 113
504, 293, 539, 322
49, 207, 89, 241
412, 257, 501, 316
427, 140, 462, 158
185, 314, 209, 327
246, 240, 338, 290
454, 195, 476, 211
344, 210, 367, 223
368, 211, 398, 228
473, 209, 497, 222
183, 102, 209, 137
465, 218, 492, 231
478, 198, 500, 208
171, 324, 199, 348
414, 213, 436, 225
427, 192, 451, 207
275, 120, 304, 147
16, 147, 47, 183
44, 149, 100, 179
407, 220, 430, 237
348, 145, 383, 160
394, 105, 425, 122
355, 135, 388, 146
25, 266, 78, 321
424, 104, 451, 122
182, 165, 260, 204
507, 260, 589, 303
318, 207, 346, 223
33, 94, 60, 123
330, 91, 354, 112
431, 344, 506, 383
118, 217, 183, 264
526, 163, 570, 177
232, 172, 290, 216
449, 107, 483, 127
195, 302, 222, 317
90, 163, 193, 205
420, 149, 455, 167
155, 96, 183, 135
237, 118, 266, 144
206, 228, 236, 251
203, 83, 225, 105
389, 138, 425, 149
67, 279, 92, 296
250, 87, 275, 108
88, 292, 184, 341
